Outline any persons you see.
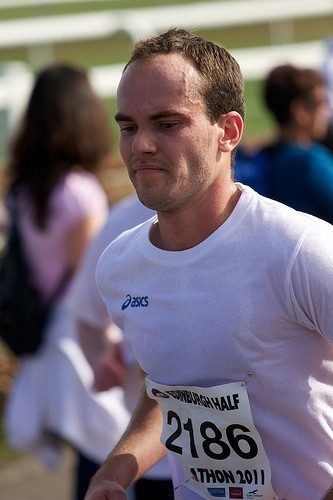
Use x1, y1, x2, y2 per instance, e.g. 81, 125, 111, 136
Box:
4, 188, 175, 500
82, 26, 331, 500
233, 40, 332, 221
0, 60, 108, 313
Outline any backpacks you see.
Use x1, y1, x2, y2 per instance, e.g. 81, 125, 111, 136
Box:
1, 214, 67, 357
